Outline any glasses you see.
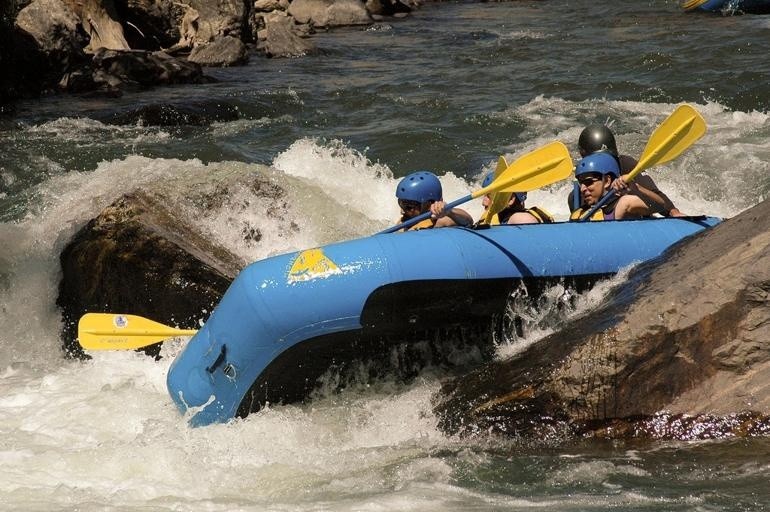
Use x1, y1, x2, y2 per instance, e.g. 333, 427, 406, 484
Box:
576, 176, 598, 189
399, 200, 418, 217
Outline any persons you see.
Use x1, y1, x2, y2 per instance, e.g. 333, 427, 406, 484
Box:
482, 169, 541, 226
569, 153, 664, 223
568, 123, 684, 218
390, 170, 474, 231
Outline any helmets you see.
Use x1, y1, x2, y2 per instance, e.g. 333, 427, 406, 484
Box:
578, 126, 620, 160
483, 171, 527, 208
574, 153, 622, 181
396, 172, 441, 209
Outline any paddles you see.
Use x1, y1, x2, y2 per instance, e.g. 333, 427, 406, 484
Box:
579, 104, 708, 222
484, 156, 513, 225
78, 312, 199, 352
371, 141, 573, 236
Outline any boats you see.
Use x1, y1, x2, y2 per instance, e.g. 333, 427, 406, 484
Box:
167, 213, 745, 430
